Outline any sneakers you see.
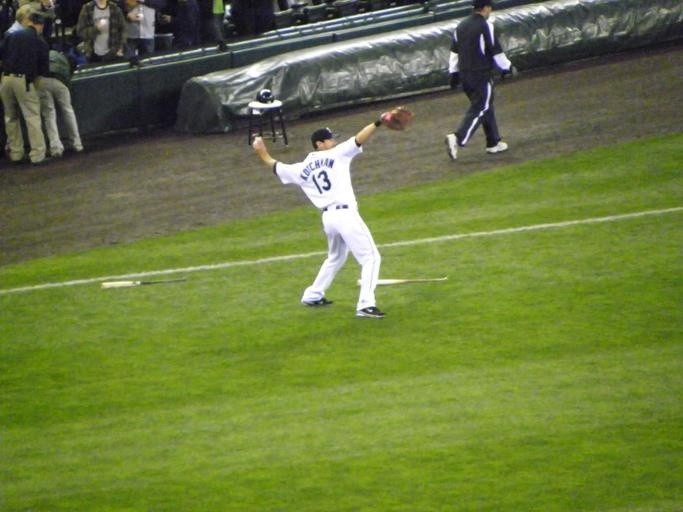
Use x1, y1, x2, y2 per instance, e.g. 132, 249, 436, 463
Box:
356, 307, 386, 318
74, 145, 84, 152
302, 298, 331, 306
445, 134, 459, 160
33, 158, 53, 166
51, 150, 62, 158
488, 142, 508, 153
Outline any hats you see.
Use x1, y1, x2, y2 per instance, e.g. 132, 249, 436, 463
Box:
15, 4, 56, 23
311, 128, 339, 139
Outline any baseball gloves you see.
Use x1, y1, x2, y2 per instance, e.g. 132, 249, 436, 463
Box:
380, 107, 411, 130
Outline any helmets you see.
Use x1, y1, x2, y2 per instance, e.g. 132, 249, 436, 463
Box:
256, 89, 274, 102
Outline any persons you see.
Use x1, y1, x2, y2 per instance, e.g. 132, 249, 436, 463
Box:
252, 107, 412, 318
0, 12, 50, 166
34, 49, 86, 159
2, 1, 288, 65
444, 0, 519, 164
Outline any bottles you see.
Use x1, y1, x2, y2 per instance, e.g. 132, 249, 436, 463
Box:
137, 6, 145, 20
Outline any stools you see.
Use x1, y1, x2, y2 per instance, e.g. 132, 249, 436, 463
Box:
249, 100, 289, 147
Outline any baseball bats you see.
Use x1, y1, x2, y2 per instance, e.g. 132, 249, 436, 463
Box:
103, 278, 185, 289
356, 278, 447, 285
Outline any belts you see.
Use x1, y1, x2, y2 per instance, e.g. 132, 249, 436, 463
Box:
2, 72, 22, 79
323, 206, 347, 212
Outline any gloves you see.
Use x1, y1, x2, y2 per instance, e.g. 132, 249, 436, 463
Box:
502, 66, 512, 79
449, 76, 458, 90
